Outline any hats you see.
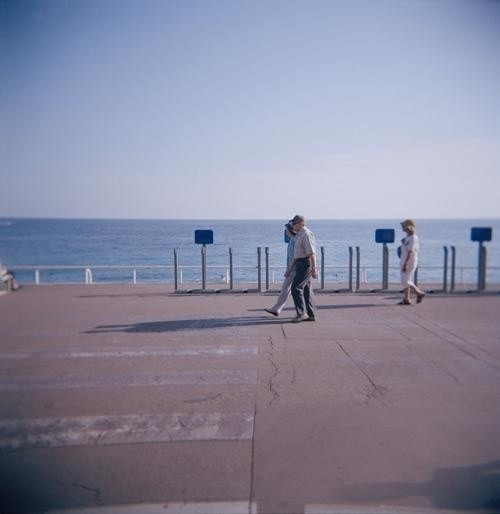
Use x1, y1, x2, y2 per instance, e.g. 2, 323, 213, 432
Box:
285, 215, 304, 229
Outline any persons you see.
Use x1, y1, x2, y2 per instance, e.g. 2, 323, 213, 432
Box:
0, 264, 20, 290
283, 215, 318, 324
397, 220, 425, 305
264, 222, 298, 316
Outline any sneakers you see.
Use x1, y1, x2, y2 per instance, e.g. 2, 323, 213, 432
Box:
417, 291, 426, 303
397, 299, 411, 304
291, 313, 316, 322
264, 304, 282, 315
